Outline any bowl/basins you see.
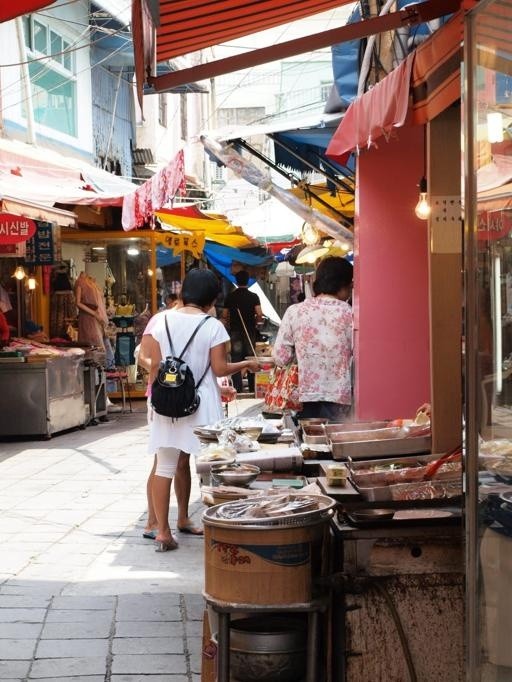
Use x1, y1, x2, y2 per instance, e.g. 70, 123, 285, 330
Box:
233, 426, 263, 441
208, 467, 261, 490
245, 355, 277, 370
208, 463, 261, 472
351, 508, 395, 520
261, 411, 283, 419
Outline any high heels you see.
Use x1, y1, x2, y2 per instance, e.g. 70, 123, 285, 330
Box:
154, 537, 179, 553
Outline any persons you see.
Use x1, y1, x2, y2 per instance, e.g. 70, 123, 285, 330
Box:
75, 271, 122, 411
164, 293, 178, 310
138, 267, 261, 552
271, 256, 353, 422
222, 271, 262, 392
134, 283, 191, 539
115, 293, 138, 315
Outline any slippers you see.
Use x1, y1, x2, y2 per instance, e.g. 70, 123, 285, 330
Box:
177, 522, 205, 536
143, 528, 160, 540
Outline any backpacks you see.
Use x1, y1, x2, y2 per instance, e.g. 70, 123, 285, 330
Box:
150, 311, 212, 419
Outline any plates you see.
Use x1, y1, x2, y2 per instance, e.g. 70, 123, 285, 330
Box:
482, 458, 512, 477
499, 490, 512, 504
200, 485, 259, 500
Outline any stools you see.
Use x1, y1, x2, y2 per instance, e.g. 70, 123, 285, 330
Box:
103, 358, 131, 414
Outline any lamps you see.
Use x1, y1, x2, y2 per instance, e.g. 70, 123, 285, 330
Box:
414, 118, 433, 220
27, 263, 37, 291
14, 256, 25, 280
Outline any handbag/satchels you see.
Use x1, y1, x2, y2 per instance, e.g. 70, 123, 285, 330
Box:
263, 356, 303, 413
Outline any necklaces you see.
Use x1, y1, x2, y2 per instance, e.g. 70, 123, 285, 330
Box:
183, 305, 208, 313
